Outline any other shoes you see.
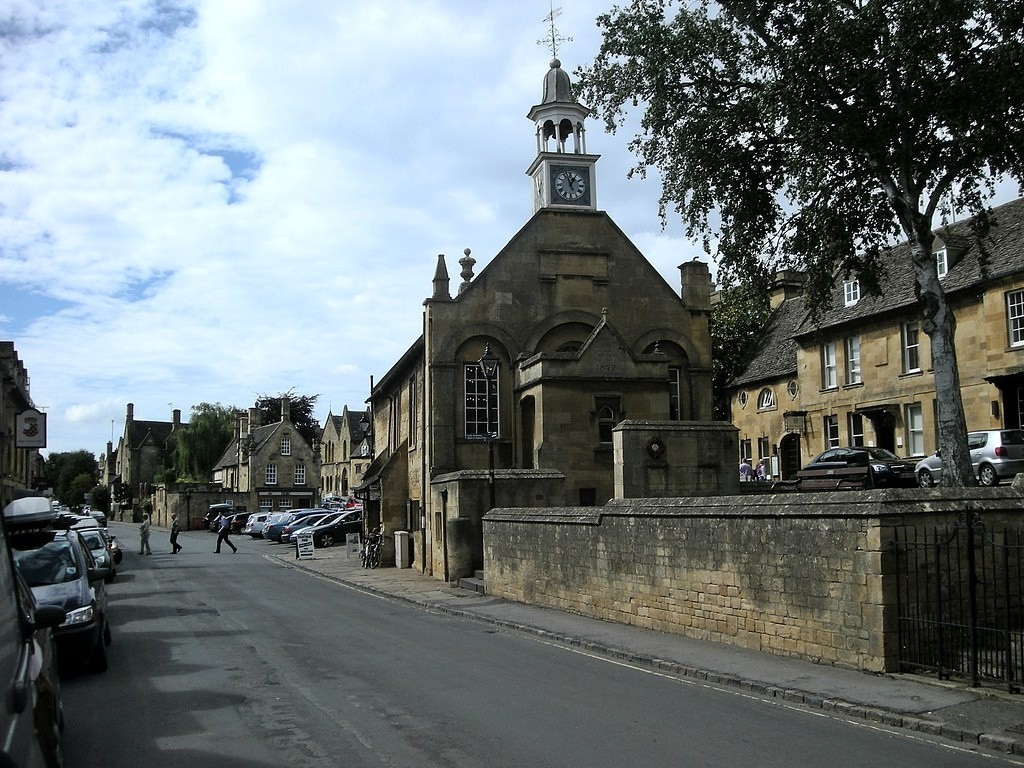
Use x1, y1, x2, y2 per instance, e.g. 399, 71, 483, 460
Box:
170, 552, 177, 554
233, 548, 237, 554
213, 551, 220, 553
138, 552, 144, 554
178, 547, 182, 552
146, 552, 152, 555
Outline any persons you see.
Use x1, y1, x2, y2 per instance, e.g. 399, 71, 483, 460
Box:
214, 511, 237, 553
757, 459, 767, 481
138, 513, 152, 555
170, 512, 182, 554
739, 458, 751, 475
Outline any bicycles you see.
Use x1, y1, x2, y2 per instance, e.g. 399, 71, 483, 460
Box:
358, 527, 384, 570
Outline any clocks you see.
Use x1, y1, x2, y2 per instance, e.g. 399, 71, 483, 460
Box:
555, 170, 585, 201
534, 172, 544, 198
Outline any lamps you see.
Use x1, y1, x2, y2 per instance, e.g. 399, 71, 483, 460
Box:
359, 414, 372, 438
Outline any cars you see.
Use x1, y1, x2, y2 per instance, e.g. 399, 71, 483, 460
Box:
802, 446, 917, 489
202, 495, 363, 548
914, 429, 1024, 489
0, 496, 123, 768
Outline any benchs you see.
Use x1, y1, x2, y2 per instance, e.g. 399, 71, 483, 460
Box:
770, 467, 869, 494
